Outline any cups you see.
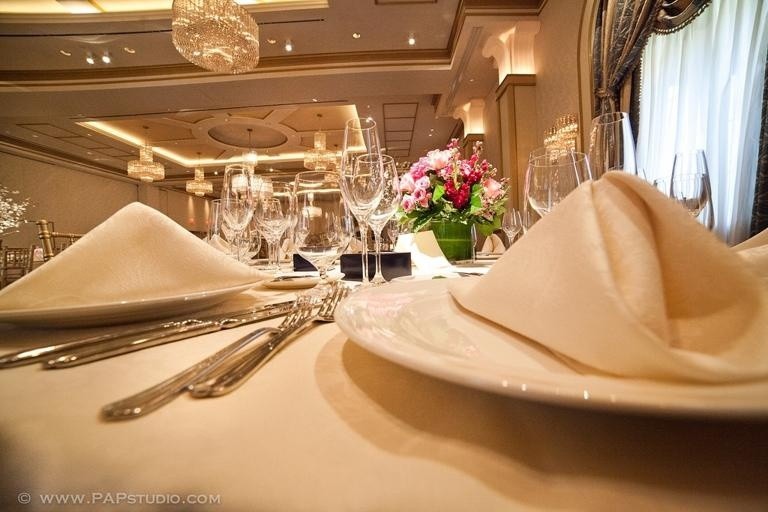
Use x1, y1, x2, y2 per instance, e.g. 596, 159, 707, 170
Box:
522, 110, 714, 239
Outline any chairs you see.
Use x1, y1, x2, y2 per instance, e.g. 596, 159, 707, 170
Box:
0, 218, 85, 290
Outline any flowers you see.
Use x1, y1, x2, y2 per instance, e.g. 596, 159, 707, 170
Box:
392, 138, 511, 236
0, 184, 38, 236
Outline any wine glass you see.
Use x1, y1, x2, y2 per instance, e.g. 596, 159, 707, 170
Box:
207, 118, 402, 291
502, 210, 522, 248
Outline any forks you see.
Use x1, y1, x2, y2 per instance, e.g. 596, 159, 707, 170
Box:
99, 283, 353, 423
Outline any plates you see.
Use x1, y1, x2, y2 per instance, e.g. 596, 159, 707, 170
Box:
0, 280, 260, 331
333, 276, 768, 424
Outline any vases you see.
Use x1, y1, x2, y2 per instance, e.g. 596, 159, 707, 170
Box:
429, 221, 473, 261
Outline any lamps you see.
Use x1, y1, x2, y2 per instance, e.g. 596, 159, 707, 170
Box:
482, 21, 541, 86
171, 1, 260, 76
452, 98, 486, 139
126, 114, 339, 197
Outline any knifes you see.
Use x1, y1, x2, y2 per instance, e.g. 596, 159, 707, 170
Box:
0, 296, 324, 372
